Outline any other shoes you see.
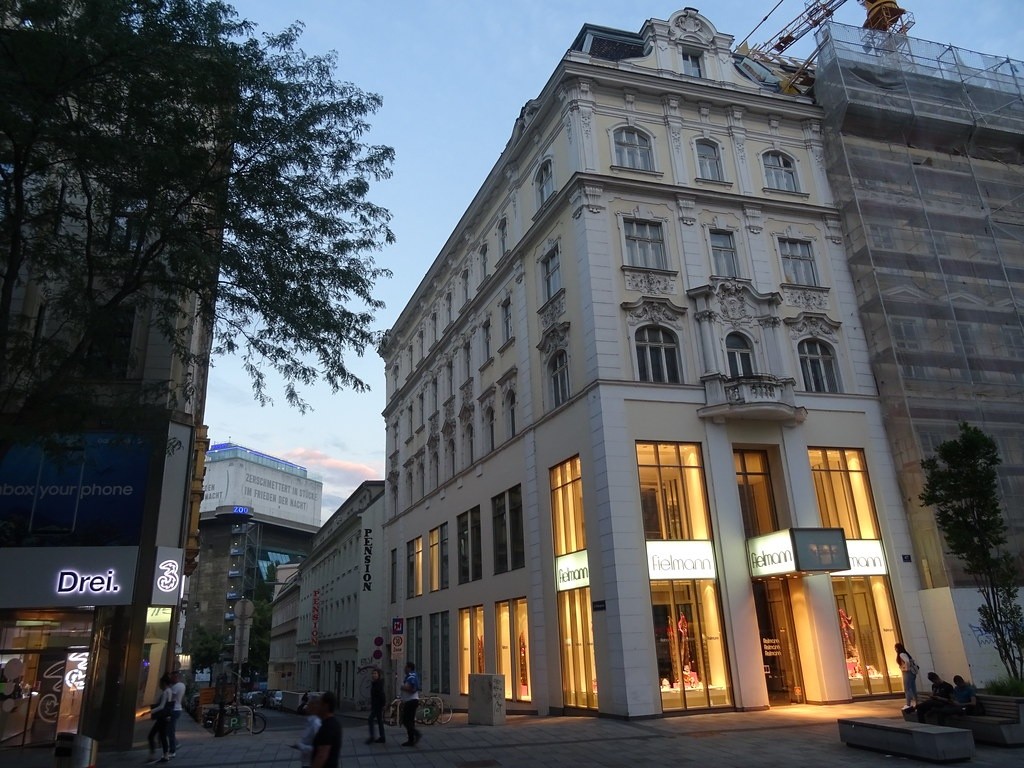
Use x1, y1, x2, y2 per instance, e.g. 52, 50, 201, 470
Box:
415, 733, 422, 745
901, 704, 913, 710
176, 743, 182, 750
161, 752, 176, 758
401, 740, 415, 747
365, 737, 375, 745
913, 703, 918, 707
147, 758, 157, 765
373, 736, 385, 744
155, 758, 168, 763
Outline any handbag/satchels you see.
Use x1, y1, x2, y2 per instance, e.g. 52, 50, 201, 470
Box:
926, 708, 936, 717
965, 701, 986, 716
150, 691, 176, 721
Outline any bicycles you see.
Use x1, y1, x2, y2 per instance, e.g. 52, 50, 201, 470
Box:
382, 694, 453, 727
213, 698, 269, 736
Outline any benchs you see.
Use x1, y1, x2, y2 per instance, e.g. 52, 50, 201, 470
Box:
902, 692, 1024, 748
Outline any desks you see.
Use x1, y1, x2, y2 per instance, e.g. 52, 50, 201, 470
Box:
837, 717, 977, 764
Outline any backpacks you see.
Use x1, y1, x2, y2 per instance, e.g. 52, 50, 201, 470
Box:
899, 651, 918, 674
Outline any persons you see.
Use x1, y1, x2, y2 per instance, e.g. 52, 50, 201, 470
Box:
135, 671, 186, 765
365, 669, 386, 744
399, 662, 422, 747
290, 692, 342, 768
895, 643, 919, 710
936, 676, 976, 726
916, 672, 954, 725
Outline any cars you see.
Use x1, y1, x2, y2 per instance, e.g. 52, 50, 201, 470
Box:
239, 688, 286, 710
